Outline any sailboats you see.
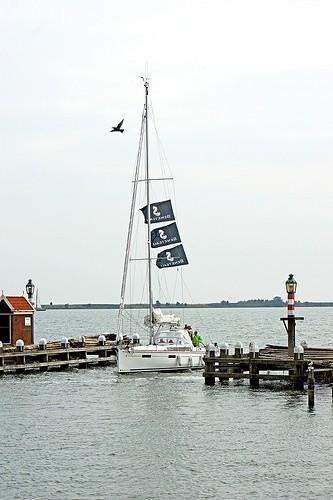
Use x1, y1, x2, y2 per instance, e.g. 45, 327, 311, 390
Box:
117, 74, 204, 376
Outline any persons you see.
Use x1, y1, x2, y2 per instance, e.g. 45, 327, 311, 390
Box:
169, 340, 173, 343
191, 330, 202, 347
187, 326, 193, 341
184, 324, 188, 329
159, 339, 165, 343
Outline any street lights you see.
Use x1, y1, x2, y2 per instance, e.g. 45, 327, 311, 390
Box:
25, 279, 35, 305
285, 274, 297, 357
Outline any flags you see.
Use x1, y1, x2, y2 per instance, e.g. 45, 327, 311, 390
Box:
156, 243, 188, 268
150, 222, 180, 247
140, 199, 175, 224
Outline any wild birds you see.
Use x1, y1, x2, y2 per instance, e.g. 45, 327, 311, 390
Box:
110, 119, 124, 133
